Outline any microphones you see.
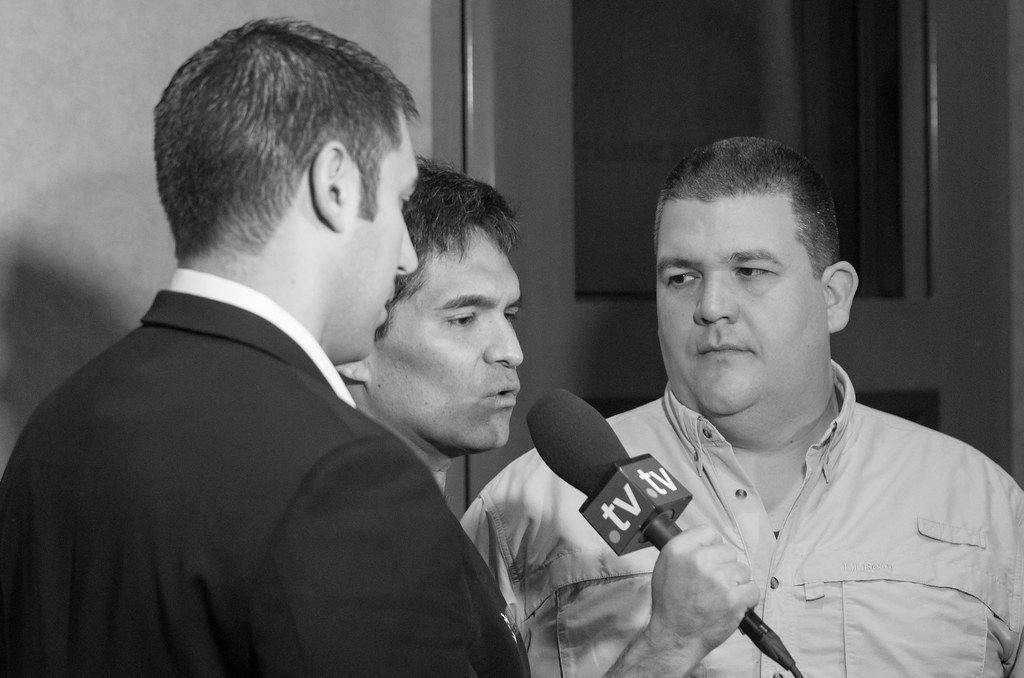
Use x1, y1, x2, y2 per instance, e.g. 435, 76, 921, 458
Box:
526, 388, 796, 670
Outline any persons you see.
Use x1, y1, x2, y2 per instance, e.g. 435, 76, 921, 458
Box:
0, 17, 537, 678
335, 154, 764, 678
460, 134, 1024, 678
